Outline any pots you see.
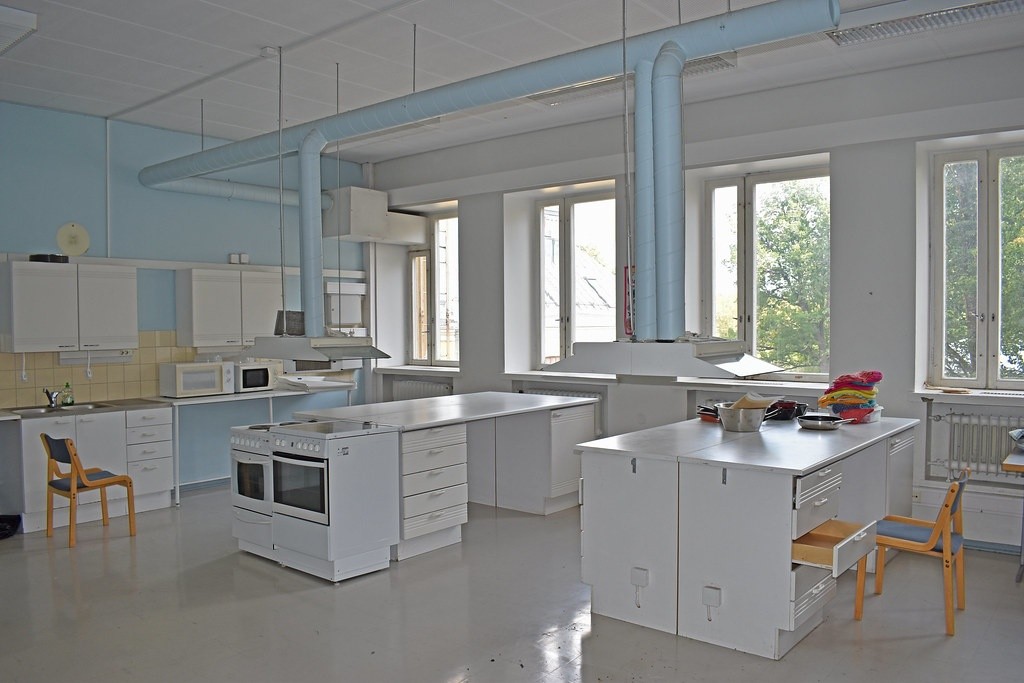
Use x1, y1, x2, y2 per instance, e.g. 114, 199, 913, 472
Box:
797, 416, 857, 430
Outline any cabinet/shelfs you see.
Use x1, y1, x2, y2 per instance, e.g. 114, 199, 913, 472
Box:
176, 267, 284, 349
774, 426, 914, 661
16, 405, 175, 534
0, 258, 140, 353
543, 403, 595, 516
396, 425, 469, 560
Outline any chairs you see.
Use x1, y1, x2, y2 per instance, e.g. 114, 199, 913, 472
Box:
39, 433, 136, 548
850, 468, 969, 635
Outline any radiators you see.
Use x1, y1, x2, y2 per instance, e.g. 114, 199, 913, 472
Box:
942, 412, 1024, 478
392, 379, 451, 400
525, 389, 601, 401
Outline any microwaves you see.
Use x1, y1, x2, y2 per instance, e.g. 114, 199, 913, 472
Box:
234, 361, 283, 392
159, 361, 234, 398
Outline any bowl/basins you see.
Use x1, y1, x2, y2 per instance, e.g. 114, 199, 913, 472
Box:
714, 402, 768, 432
766, 401, 808, 420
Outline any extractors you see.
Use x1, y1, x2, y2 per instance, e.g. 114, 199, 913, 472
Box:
536, 340, 785, 378
245, 337, 391, 362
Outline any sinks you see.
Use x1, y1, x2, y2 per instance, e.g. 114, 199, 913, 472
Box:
10, 408, 48, 415
61, 405, 95, 411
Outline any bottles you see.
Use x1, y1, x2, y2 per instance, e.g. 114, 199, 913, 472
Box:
61, 383, 74, 406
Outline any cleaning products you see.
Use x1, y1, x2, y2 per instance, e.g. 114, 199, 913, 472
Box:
61, 382, 75, 406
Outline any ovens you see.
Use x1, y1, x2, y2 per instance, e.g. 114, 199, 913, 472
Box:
229, 421, 400, 582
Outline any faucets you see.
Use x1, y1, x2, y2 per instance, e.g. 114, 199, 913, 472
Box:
42, 387, 64, 408
